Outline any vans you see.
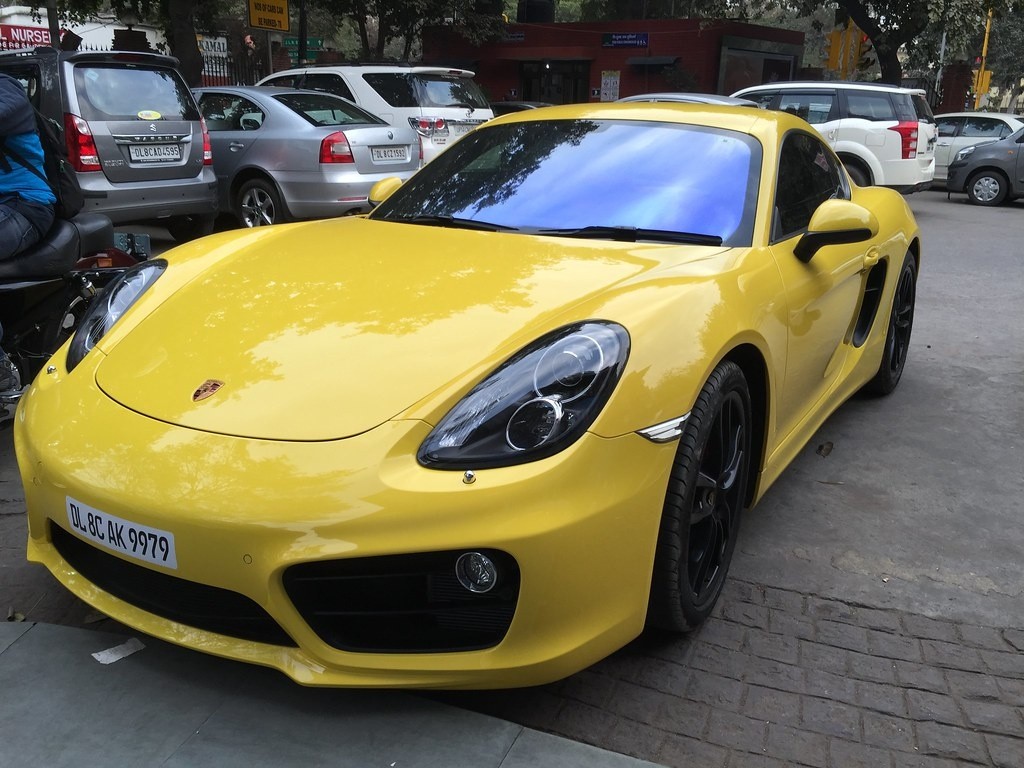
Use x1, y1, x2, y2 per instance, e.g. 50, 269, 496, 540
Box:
0, 46, 222, 230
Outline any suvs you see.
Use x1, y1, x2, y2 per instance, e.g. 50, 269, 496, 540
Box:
250, 61, 497, 172
728, 81, 939, 196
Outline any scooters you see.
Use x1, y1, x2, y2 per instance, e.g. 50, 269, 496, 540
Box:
0, 212, 149, 424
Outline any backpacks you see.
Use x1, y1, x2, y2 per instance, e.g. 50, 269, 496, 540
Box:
0, 104, 84, 221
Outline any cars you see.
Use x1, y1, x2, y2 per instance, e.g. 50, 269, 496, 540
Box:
921, 111, 1024, 207
183, 83, 424, 227
609, 92, 763, 108
489, 100, 558, 116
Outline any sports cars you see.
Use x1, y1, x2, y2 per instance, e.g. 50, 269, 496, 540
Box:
14, 102, 923, 694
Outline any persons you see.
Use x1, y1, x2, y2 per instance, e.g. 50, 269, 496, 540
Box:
0, 74, 57, 393
